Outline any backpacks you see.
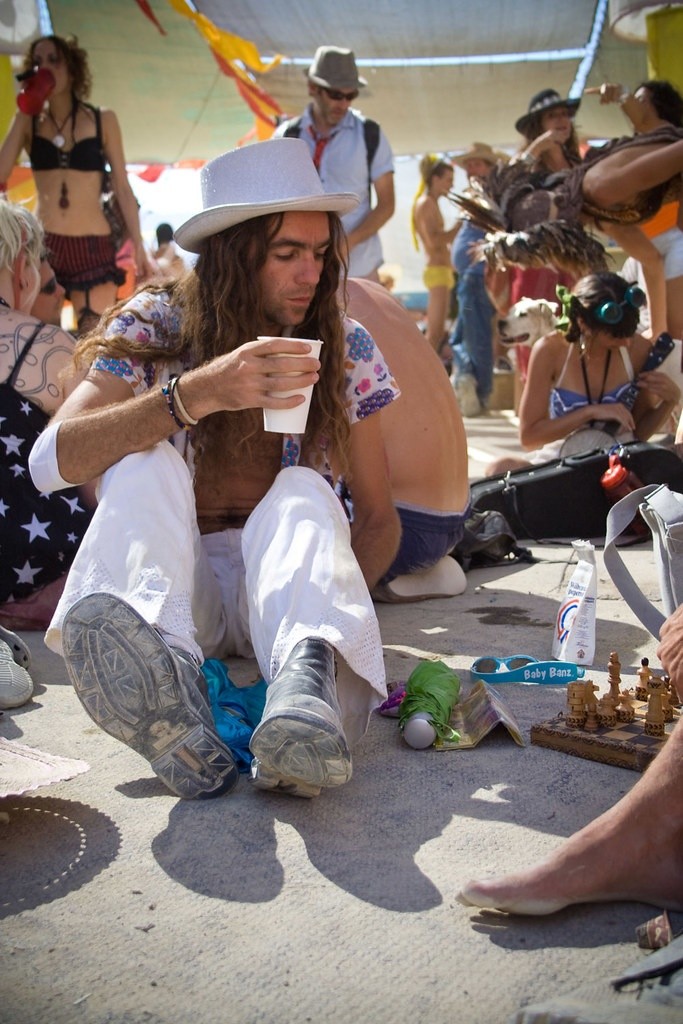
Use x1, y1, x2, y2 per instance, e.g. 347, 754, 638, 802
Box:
450, 509, 541, 574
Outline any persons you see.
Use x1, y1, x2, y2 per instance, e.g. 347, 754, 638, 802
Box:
410, 156, 462, 354
29, 137, 403, 798
454, 601, 683, 916
0, 36, 152, 330
450, 80, 683, 422
0, 201, 98, 606
149, 224, 175, 266
488, 271, 683, 478
272, 46, 394, 282
337, 278, 469, 600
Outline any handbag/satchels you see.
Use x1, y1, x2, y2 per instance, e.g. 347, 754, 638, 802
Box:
602, 484, 683, 643
552, 539, 598, 666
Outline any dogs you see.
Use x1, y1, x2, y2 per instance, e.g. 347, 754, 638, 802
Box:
498, 296, 683, 445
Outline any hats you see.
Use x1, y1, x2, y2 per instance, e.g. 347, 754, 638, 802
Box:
303, 44, 367, 90
449, 142, 497, 170
514, 88, 581, 141
411, 153, 443, 251
172, 137, 361, 253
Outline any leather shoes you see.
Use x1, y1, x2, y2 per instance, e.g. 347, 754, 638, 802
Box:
61, 592, 238, 801
250, 637, 354, 799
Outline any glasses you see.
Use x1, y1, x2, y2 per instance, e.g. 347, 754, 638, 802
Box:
39, 276, 58, 295
591, 287, 647, 324
468, 655, 586, 686
31, 51, 67, 67
321, 85, 359, 101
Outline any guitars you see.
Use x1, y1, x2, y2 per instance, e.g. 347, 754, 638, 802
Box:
559, 331, 676, 458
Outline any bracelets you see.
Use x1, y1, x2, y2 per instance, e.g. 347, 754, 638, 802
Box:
172, 378, 198, 425
162, 380, 191, 430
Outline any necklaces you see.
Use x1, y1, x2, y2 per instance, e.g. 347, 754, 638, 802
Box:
57, 146, 69, 207
48, 111, 72, 147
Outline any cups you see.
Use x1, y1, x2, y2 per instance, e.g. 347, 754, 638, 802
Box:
261, 337, 325, 435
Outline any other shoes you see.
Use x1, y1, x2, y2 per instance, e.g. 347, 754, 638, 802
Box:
0, 625, 31, 670
0, 639, 34, 709
458, 375, 481, 418
370, 555, 467, 604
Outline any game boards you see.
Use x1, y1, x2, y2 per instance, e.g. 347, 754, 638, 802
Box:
531, 681, 683, 773
